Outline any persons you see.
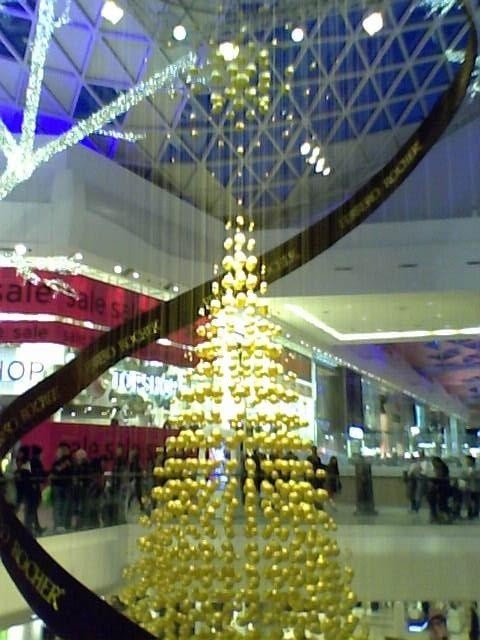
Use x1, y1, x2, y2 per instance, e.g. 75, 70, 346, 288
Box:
404, 454, 479, 522
419, 598, 479, 640
